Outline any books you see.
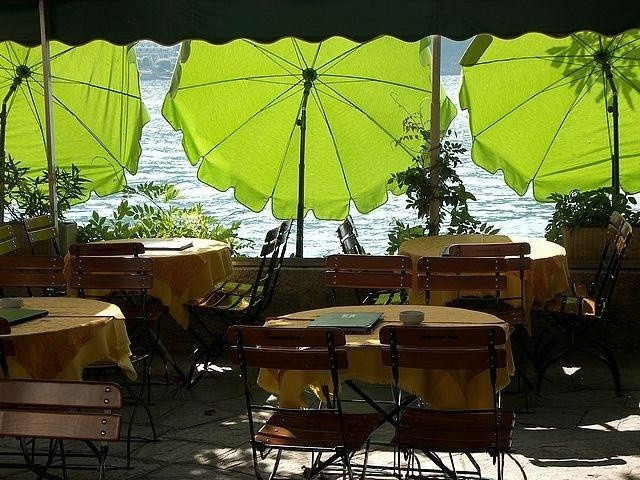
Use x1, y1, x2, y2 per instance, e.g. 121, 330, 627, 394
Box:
306, 311, 385, 335
143, 239, 193, 251
0, 306, 48, 327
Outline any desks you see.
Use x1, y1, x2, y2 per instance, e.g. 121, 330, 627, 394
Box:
398, 235, 570, 389
1, 294, 136, 462
256, 305, 516, 479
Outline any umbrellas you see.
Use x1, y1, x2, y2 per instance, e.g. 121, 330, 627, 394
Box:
0, 40, 151, 224
458, 28, 640, 214
160, 35, 456, 259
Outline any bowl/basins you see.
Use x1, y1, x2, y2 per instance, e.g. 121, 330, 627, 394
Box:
399, 309, 424, 328
0, 297, 27, 308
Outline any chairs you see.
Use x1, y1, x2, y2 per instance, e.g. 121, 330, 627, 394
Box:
2, 254, 65, 470
336, 215, 408, 305
416, 255, 509, 317
181, 217, 292, 391
323, 253, 412, 306
530, 210, 632, 399
379, 325, 516, 480
228, 325, 381, 480
441, 242, 531, 414
2, 214, 233, 386
0, 379, 127, 478
51, 257, 162, 470
